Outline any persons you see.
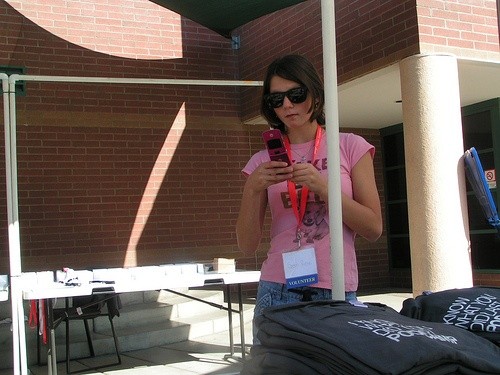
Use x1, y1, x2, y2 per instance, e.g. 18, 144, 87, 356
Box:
235, 54, 382, 347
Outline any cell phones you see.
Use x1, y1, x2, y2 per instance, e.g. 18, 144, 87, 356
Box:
263, 129, 292, 168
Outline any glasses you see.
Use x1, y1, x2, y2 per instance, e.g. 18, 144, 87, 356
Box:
264, 86, 308, 108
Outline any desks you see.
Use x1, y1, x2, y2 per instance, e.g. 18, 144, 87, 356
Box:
23, 269, 261, 375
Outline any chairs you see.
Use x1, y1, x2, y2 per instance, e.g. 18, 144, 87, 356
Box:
36, 294, 122, 375
